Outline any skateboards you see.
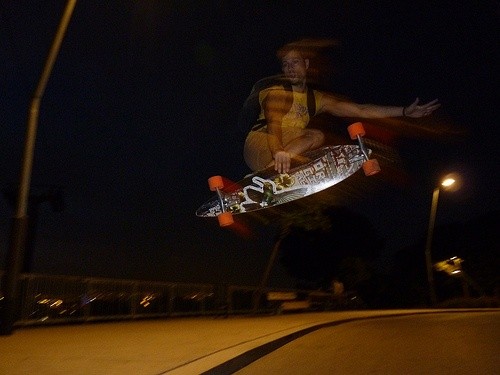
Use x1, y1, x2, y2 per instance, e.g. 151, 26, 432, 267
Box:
195, 122, 381, 227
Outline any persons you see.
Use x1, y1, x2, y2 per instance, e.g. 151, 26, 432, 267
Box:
243, 43, 442, 175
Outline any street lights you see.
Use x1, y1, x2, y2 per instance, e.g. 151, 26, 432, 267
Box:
425, 173, 455, 307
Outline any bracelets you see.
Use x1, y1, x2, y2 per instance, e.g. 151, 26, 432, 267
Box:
403, 103, 408, 122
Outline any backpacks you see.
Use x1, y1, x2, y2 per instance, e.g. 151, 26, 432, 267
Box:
236, 79, 314, 142
